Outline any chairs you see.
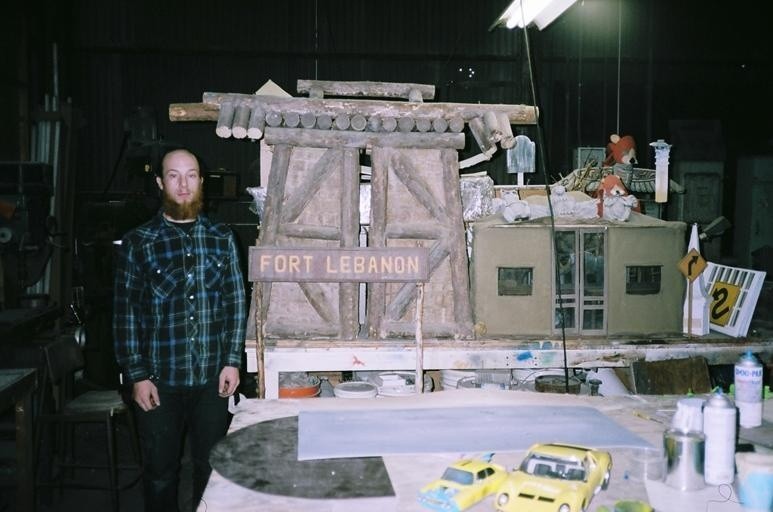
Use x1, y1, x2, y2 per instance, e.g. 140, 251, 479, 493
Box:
31, 334, 148, 511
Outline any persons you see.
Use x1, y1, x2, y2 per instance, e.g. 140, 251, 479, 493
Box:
113, 148, 247, 512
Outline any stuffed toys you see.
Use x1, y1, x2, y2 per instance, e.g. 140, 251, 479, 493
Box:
596, 174, 639, 222
602, 134, 636, 164
